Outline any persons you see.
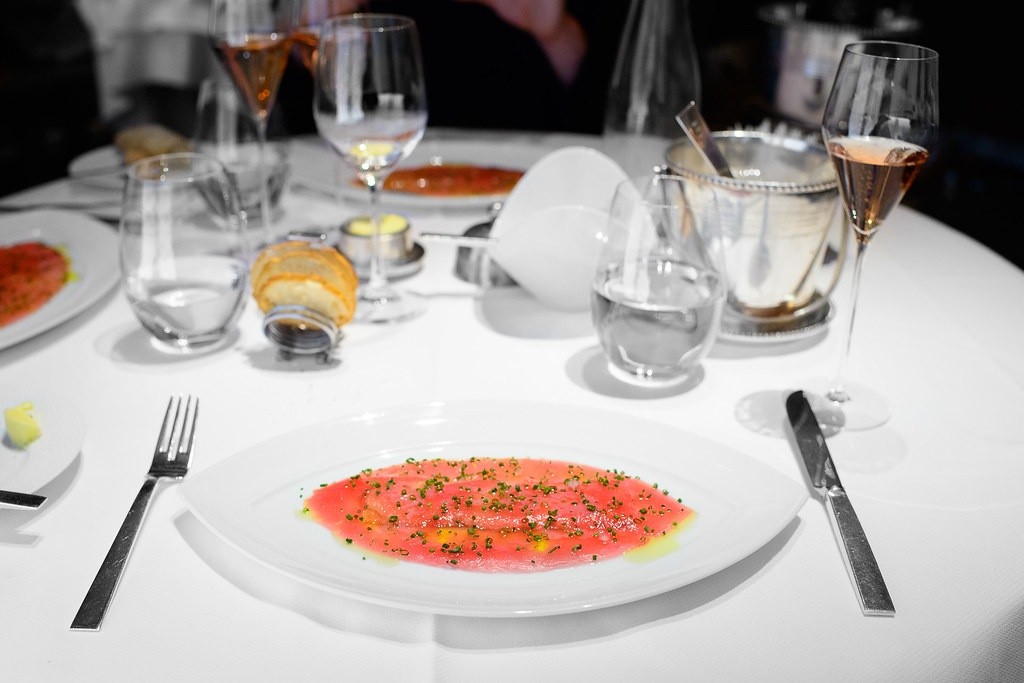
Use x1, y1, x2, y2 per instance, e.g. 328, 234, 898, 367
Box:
262, 0, 688, 139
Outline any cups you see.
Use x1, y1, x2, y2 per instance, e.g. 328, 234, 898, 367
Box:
194, 77, 288, 224
594, 173, 726, 388
660, 128, 853, 324
118, 151, 250, 353
479, 143, 657, 314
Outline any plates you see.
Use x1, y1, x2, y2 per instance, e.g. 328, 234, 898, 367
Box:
0, 206, 125, 350
0, 383, 89, 509
298, 138, 561, 214
709, 290, 837, 341
69, 135, 233, 205
183, 403, 812, 618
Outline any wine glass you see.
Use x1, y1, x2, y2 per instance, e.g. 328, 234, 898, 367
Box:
311, 13, 430, 324
205, 0, 373, 247
794, 39, 941, 434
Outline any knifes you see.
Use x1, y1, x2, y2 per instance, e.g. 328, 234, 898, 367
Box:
782, 389, 895, 617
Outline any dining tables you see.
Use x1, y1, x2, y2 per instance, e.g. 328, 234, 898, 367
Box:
0, 132, 1024, 683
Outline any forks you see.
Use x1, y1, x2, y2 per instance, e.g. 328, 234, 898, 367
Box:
69, 391, 201, 632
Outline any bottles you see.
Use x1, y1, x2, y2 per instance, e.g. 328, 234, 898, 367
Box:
597, 0, 706, 186
